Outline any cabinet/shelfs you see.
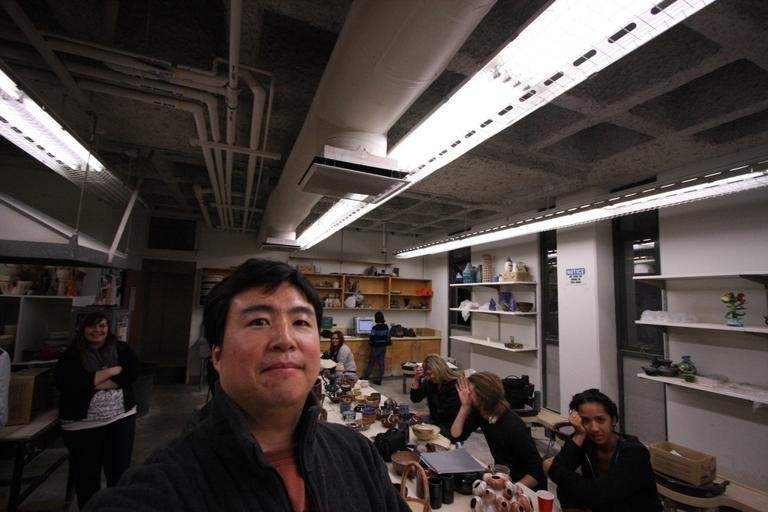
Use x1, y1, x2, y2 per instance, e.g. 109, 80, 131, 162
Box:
632, 270, 768, 404
448, 280, 537, 353
298, 271, 433, 312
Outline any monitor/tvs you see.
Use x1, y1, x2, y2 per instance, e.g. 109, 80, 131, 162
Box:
147, 215, 197, 252
353, 317, 375, 335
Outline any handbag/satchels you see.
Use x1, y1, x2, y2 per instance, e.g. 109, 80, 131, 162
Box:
374, 428, 405, 462
502, 374, 534, 408
400, 461, 432, 512
391, 325, 404, 337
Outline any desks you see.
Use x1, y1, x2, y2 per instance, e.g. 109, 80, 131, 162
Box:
313, 368, 562, 511
0, 402, 75, 512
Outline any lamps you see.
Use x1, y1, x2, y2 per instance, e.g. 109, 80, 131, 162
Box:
297, 0, 716, 252
393, 160, 768, 261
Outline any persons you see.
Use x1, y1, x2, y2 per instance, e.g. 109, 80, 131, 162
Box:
79, 258, 413, 512
328, 330, 358, 372
359, 312, 390, 385
409, 353, 465, 439
548, 388, 666, 511
54, 310, 143, 507
449, 370, 548, 493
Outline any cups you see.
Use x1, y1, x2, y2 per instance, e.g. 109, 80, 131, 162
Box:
416, 470, 454, 509
536, 490, 555, 512
534, 391, 541, 411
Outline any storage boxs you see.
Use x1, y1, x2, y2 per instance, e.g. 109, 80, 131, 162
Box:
6, 367, 49, 427
649, 440, 718, 487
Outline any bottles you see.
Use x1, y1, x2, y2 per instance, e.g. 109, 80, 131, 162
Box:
504, 255, 512, 272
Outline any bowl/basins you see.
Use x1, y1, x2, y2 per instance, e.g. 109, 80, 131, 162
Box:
0, 281, 32, 295
391, 450, 421, 477
411, 423, 441, 440
515, 301, 533, 312
